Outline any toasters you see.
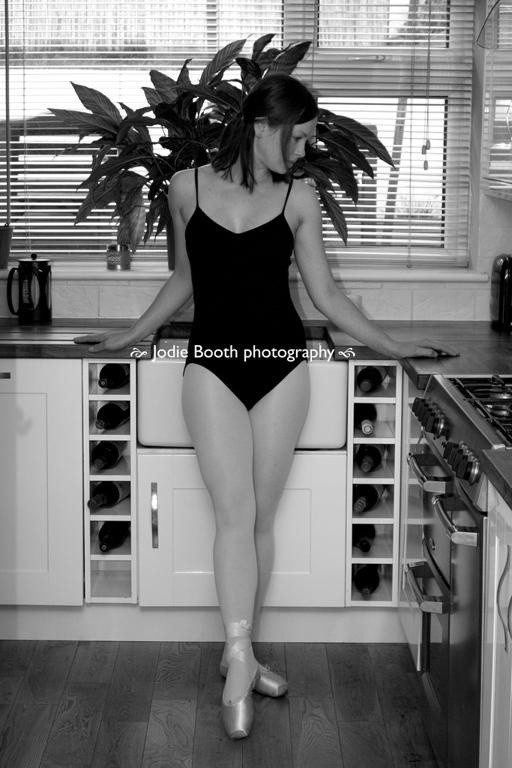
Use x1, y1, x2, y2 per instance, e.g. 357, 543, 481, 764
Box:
488, 253, 511, 329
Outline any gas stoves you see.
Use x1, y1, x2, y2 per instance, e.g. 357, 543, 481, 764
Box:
408, 369, 512, 514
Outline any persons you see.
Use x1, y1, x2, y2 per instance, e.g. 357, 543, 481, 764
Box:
74, 74, 459, 741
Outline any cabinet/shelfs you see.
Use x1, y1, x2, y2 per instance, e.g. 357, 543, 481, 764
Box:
84, 339, 137, 643
478, 453, 512, 768
345, 349, 406, 650
0, 339, 83, 645
137, 343, 349, 645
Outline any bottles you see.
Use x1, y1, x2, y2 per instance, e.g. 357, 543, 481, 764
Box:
88, 480, 130, 512
98, 364, 130, 392
88, 440, 128, 473
6, 251, 56, 327
98, 521, 132, 554
353, 366, 389, 599
106, 243, 130, 270
95, 401, 130, 430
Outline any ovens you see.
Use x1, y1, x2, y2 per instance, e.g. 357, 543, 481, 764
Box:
397, 433, 482, 768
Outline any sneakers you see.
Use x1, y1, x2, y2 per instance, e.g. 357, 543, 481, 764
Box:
220, 618, 288, 739
